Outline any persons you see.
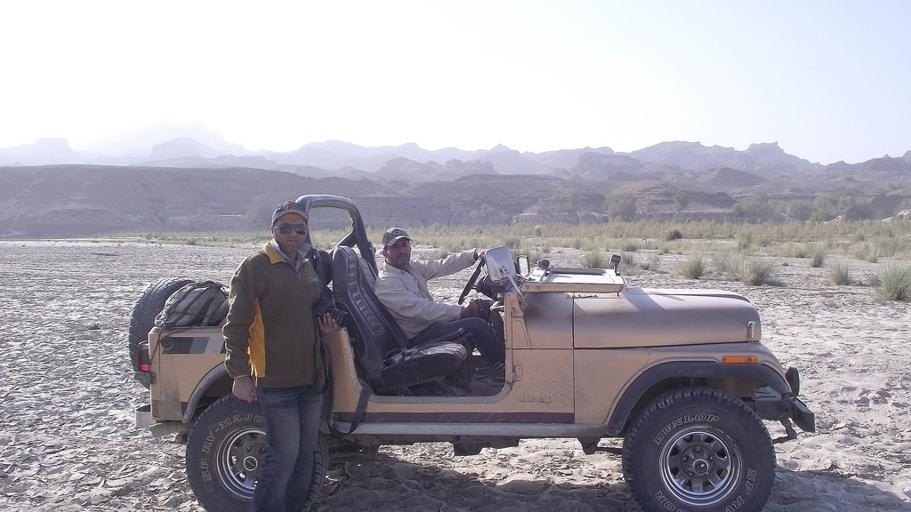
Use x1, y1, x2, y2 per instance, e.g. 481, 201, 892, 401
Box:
373, 225, 506, 366
222, 200, 341, 511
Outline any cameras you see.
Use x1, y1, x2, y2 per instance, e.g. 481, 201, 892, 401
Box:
312, 294, 349, 329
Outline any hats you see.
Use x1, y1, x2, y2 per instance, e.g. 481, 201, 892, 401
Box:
382, 228, 412, 246
271, 200, 310, 224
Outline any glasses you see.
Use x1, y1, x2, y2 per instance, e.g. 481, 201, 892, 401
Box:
275, 224, 308, 236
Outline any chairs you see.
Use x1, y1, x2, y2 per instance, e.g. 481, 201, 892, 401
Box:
326, 246, 476, 396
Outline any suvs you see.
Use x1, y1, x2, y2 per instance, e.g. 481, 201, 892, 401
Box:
121, 193, 818, 512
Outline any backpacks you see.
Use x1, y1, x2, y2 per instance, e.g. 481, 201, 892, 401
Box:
153, 280, 230, 329
